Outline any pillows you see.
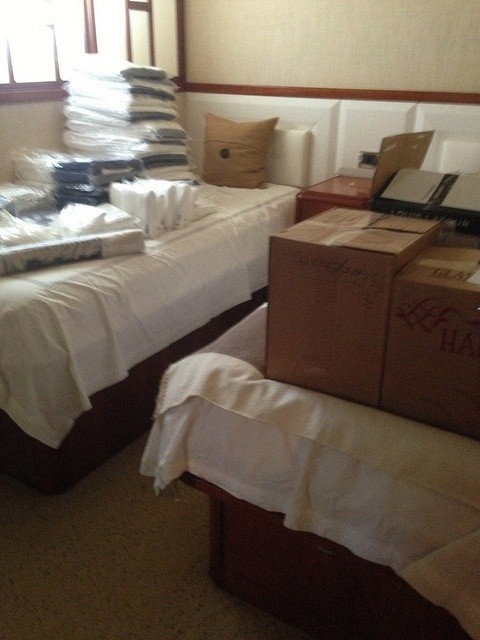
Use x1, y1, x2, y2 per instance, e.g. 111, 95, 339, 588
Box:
202, 112, 279, 189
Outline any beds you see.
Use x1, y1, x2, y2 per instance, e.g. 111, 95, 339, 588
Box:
0, 90, 340, 492
139, 301, 480, 640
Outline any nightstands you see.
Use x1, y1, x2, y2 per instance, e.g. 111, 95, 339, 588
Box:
296, 175, 372, 220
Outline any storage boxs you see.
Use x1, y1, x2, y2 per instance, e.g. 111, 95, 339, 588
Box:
381, 244, 480, 440
372, 129, 479, 237
264, 207, 442, 407
